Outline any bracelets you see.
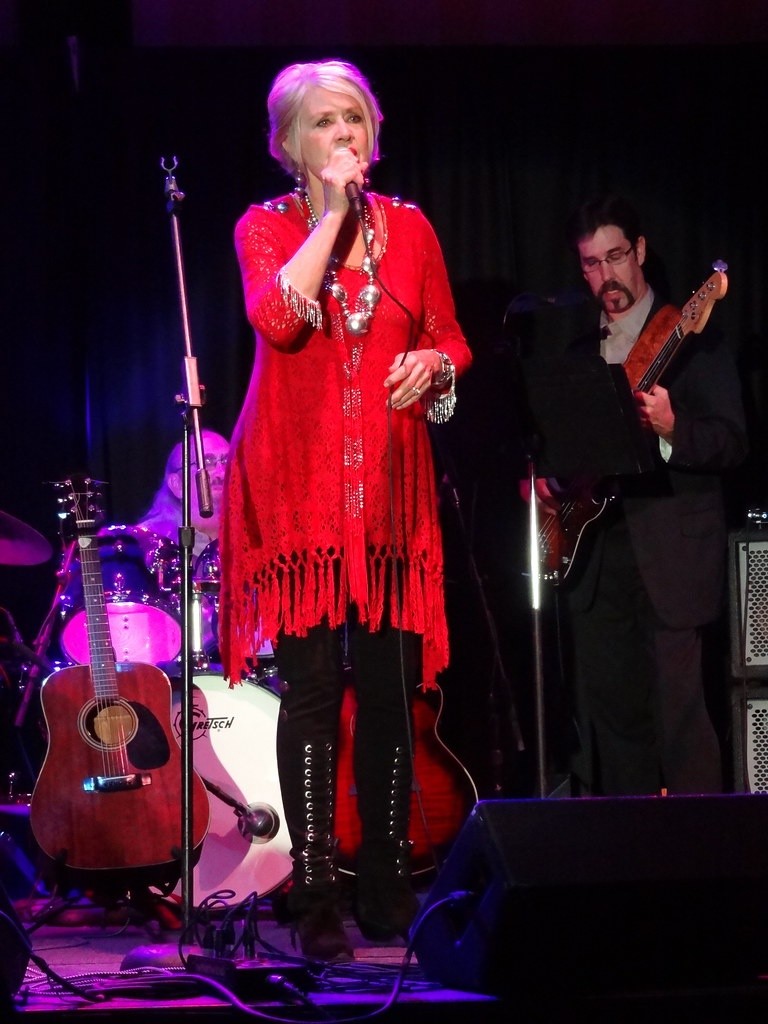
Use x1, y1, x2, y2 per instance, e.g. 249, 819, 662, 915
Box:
423, 347, 457, 424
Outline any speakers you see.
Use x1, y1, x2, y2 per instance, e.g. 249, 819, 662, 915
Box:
721, 530, 768, 793
0, 888, 33, 1001
409, 792, 768, 999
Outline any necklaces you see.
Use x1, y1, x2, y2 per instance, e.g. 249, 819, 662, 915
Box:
300, 194, 381, 517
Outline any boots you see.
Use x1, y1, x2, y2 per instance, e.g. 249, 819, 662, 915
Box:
350, 739, 422, 947
273, 733, 355, 963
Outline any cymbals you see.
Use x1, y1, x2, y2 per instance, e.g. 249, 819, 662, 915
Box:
0, 511, 54, 567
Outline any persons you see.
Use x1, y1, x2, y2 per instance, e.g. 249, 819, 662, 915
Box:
140, 429, 270, 657
509, 192, 749, 796
217, 59, 473, 961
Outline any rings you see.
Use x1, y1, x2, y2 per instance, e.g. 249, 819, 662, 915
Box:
412, 387, 420, 395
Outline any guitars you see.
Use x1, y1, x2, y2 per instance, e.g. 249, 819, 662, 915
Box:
29, 470, 211, 888
328, 663, 480, 883
518, 257, 731, 591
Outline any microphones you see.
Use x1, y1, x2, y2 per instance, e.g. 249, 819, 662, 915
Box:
336, 147, 364, 218
232, 805, 275, 836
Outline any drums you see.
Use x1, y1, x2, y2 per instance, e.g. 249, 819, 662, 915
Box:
143, 667, 299, 913
56, 521, 184, 670
191, 537, 276, 659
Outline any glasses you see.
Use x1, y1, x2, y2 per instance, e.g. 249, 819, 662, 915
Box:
175, 457, 228, 473
581, 245, 635, 273
